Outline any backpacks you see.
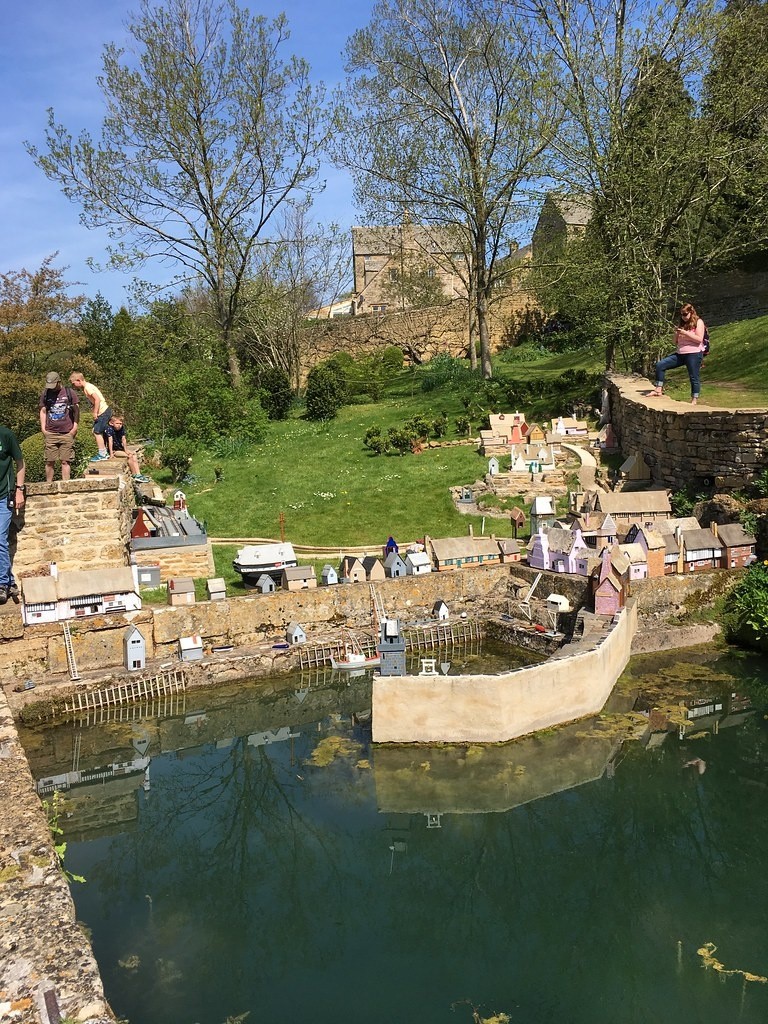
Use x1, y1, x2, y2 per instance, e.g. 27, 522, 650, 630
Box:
703, 328, 710, 355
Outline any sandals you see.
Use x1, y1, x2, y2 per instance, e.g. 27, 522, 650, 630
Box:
646, 390, 662, 397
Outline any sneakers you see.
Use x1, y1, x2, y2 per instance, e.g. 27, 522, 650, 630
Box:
134, 474, 149, 483
90, 453, 109, 461
8, 584, 19, 596
0, 587, 8, 602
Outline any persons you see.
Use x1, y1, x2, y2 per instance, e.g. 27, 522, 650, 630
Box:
68, 371, 111, 465
645, 303, 704, 405
105, 415, 150, 484
40, 372, 79, 481
0, 425, 26, 602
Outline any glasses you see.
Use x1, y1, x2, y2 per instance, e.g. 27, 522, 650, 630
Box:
681, 312, 689, 316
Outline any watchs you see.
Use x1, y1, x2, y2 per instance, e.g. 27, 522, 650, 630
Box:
16, 484, 25, 491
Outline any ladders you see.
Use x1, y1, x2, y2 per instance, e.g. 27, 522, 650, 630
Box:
72, 732, 81, 773
348, 631, 366, 659
370, 584, 382, 629
524, 573, 542, 603
63, 623, 78, 678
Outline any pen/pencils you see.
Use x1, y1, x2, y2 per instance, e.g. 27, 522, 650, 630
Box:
16, 509, 19, 515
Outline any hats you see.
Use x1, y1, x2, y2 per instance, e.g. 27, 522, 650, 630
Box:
45, 372, 61, 389
